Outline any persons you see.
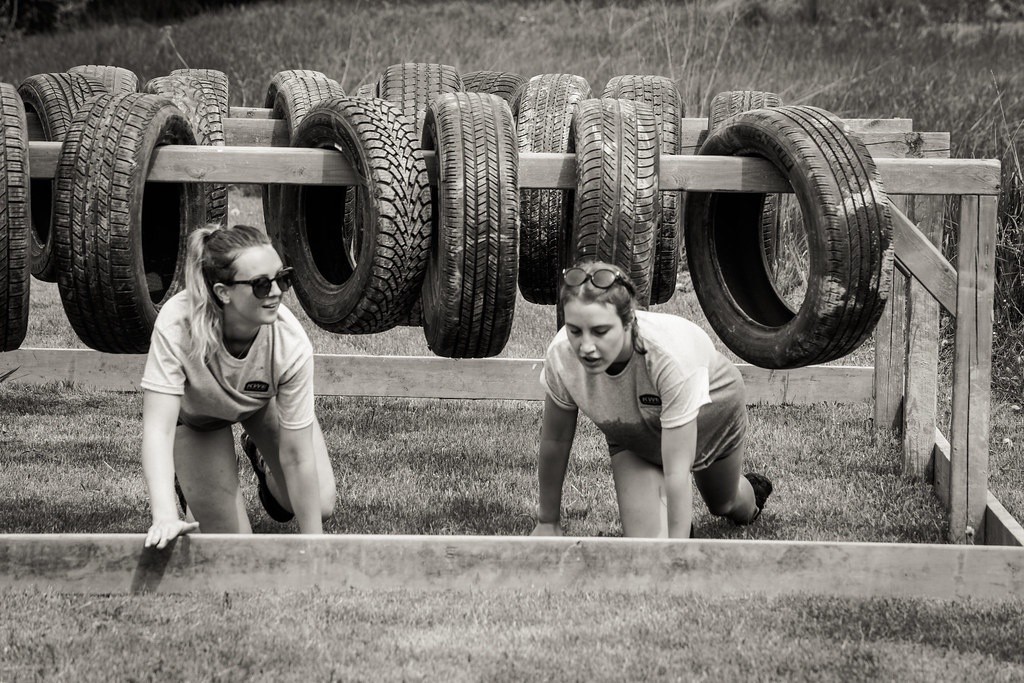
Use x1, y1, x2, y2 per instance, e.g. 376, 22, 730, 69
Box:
141, 223, 338, 549
528, 261, 774, 538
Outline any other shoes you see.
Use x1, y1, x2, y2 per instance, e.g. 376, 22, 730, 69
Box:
744, 472, 774, 526
174, 472, 189, 520
242, 431, 296, 523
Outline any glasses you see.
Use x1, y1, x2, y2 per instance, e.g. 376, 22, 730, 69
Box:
562, 267, 634, 294
225, 267, 296, 299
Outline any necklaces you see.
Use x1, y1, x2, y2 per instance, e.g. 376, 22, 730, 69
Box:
223, 332, 254, 359
